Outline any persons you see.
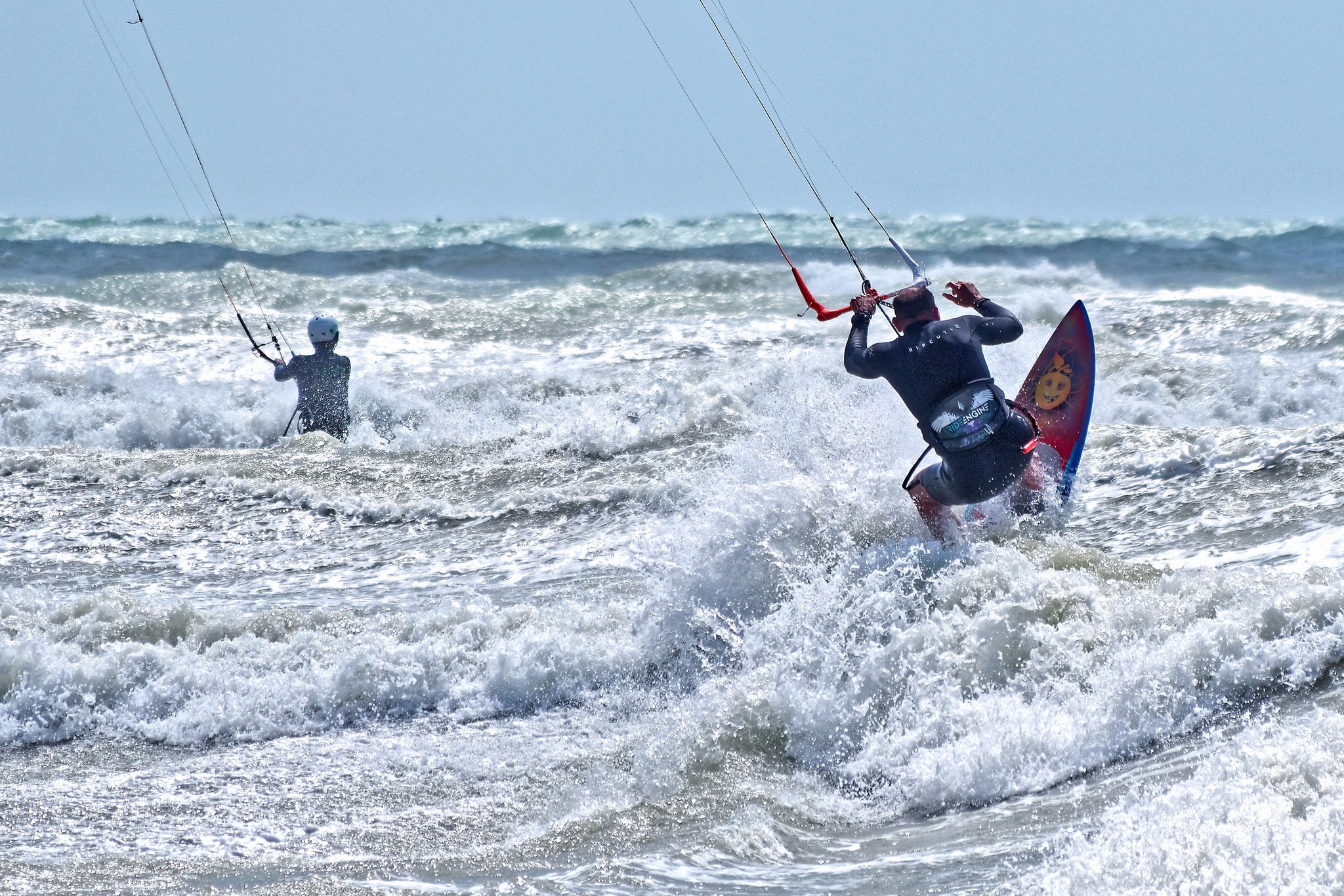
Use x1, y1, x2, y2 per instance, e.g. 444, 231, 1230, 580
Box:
843, 280, 1042, 550
274, 314, 350, 443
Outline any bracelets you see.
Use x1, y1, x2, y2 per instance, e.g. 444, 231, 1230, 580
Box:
275, 362, 284, 367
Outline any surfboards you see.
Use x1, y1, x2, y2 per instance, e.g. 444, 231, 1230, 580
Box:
964, 299, 1096, 524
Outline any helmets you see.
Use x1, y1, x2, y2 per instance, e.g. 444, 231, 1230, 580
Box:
306, 314, 338, 343
